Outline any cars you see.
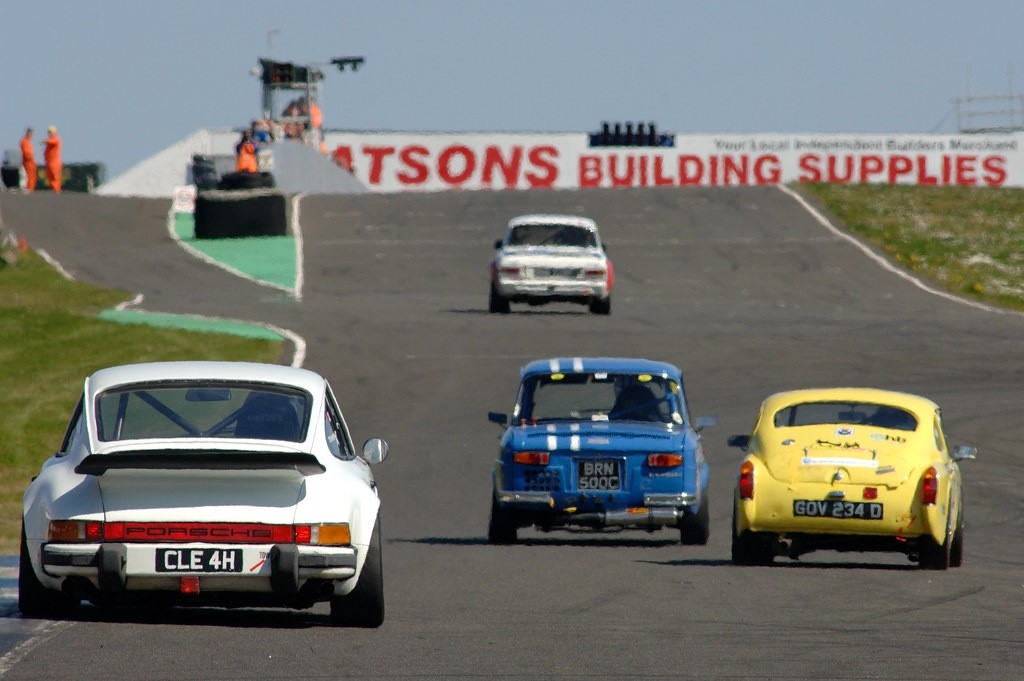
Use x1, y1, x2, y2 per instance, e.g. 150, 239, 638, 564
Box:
488, 212, 613, 316
482, 355, 712, 546
18, 360, 390, 627
726, 386, 979, 570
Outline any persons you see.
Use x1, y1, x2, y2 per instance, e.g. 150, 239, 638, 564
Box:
235, 96, 329, 175
21, 127, 38, 195
40, 125, 62, 192
